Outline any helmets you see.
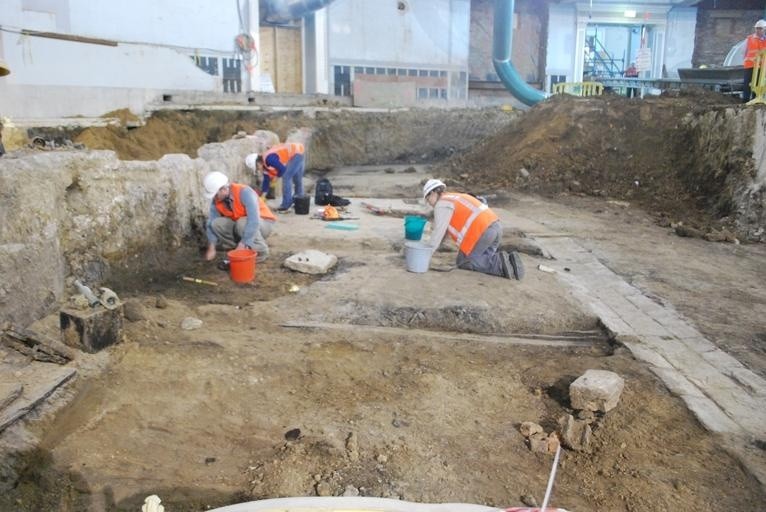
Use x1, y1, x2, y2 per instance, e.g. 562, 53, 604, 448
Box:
246, 154, 258, 175
753, 20, 766, 27
423, 179, 446, 200
203, 172, 227, 199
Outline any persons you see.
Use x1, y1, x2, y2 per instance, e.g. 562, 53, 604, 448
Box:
625, 62, 638, 98
246, 143, 304, 211
743, 19, 766, 99
423, 179, 525, 281
202, 172, 278, 263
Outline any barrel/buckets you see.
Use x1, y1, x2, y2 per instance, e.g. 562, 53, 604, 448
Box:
404, 240, 434, 273
404, 214, 428, 239
226, 249, 258, 284
293, 194, 310, 215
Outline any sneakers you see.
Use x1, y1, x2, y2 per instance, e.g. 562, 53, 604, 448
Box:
500, 250, 524, 280
